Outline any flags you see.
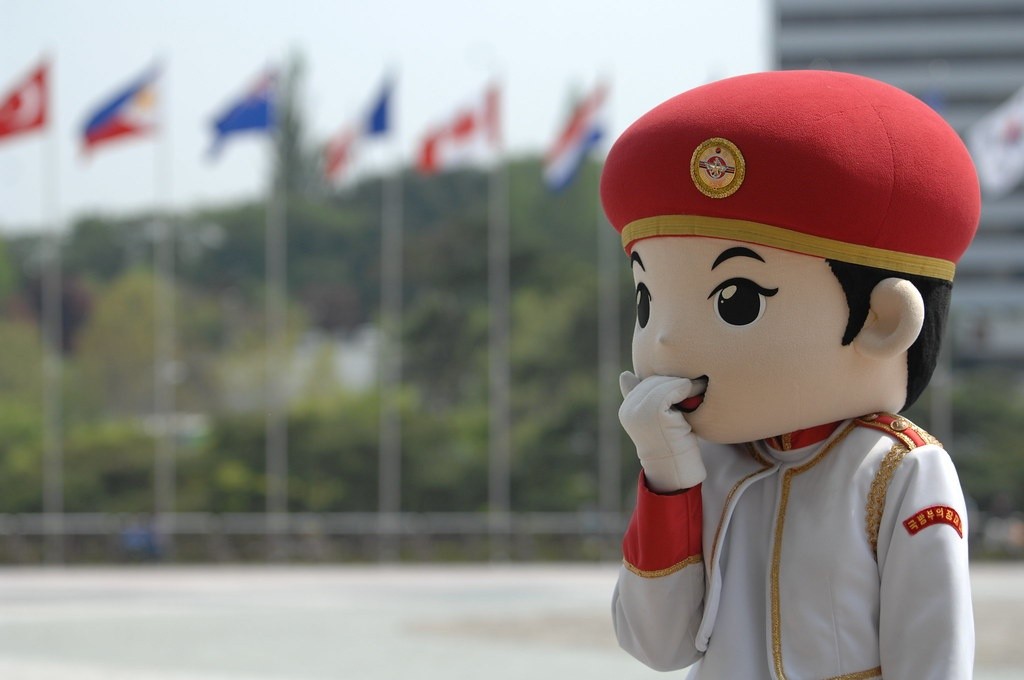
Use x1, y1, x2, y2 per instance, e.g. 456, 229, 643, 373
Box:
0, 55, 51, 134
82, 61, 165, 145
318, 74, 390, 190
966, 85, 1024, 201
213, 74, 288, 141
540, 101, 604, 193
413, 99, 509, 175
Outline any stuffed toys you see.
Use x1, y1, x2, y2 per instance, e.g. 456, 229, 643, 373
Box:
598, 68, 981, 680
